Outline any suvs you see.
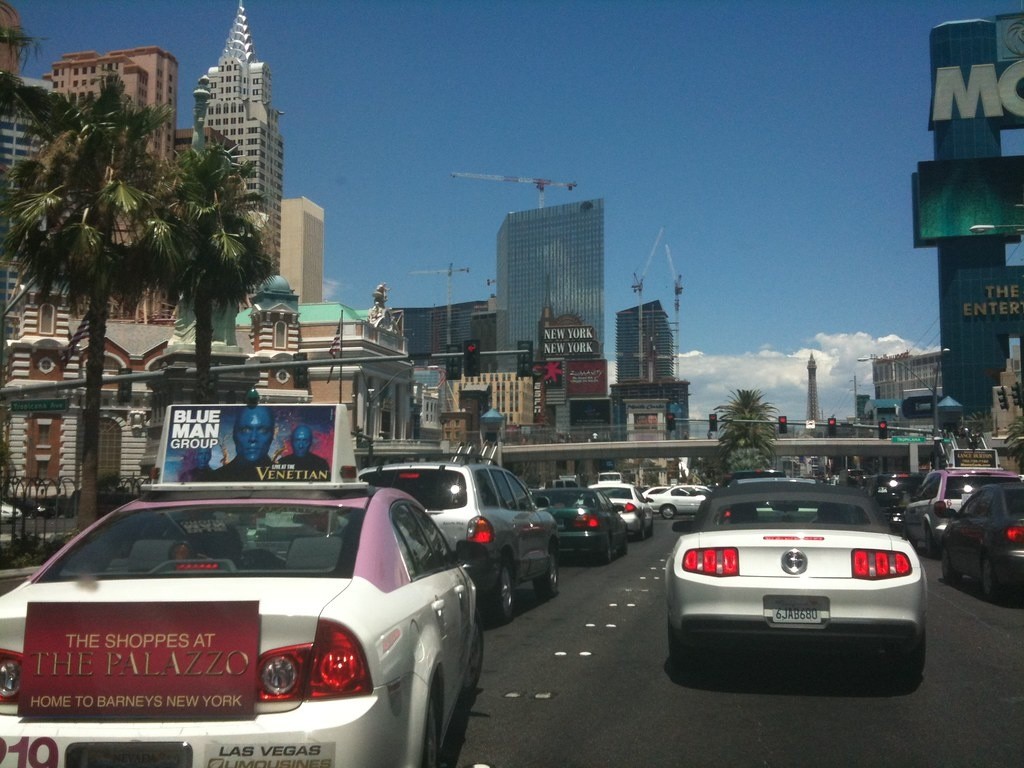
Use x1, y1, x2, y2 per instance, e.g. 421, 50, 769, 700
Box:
848, 466, 1024, 557
357, 465, 574, 628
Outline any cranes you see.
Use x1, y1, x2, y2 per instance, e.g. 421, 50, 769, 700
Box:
631, 227, 668, 383
673, 274, 686, 382
408, 262, 470, 343
451, 171, 578, 209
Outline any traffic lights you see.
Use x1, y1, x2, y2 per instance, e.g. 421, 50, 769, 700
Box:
779, 416, 788, 434
828, 418, 836, 438
1011, 385, 1020, 405
997, 390, 1006, 409
666, 414, 675, 430
878, 421, 887, 439
463, 340, 481, 377
709, 413, 718, 432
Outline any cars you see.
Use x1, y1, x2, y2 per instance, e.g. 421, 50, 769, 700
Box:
665, 468, 927, 691
0, 406, 480, 768
0, 500, 22, 524
533, 481, 711, 567
934, 480, 1024, 607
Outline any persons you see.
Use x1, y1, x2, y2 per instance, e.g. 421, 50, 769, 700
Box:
179, 447, 214, 482
210, 405, 279, 482
276, 425, 331, 482
370, 282, 391, 321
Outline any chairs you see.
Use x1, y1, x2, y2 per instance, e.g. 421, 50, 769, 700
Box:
725, 502, 761, 524
199, 523, 247, 571
109, 536, 197, 573
816, 500, 847, 524
284, 534, 344, 570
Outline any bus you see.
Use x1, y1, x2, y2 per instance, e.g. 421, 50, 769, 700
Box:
598, 471, 636, 483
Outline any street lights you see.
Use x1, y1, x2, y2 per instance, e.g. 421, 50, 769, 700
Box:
858, 347, 953, 436
